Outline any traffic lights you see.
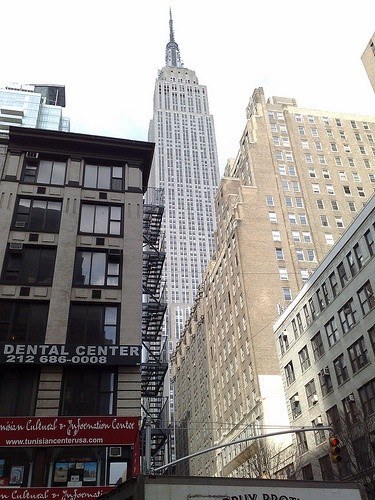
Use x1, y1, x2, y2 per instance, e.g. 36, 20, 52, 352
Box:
329, 437, 343, 463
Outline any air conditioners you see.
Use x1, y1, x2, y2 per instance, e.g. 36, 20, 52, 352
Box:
282, 332, 287, 337
24, 151, 39, 161
346, 395, 354, 402
109, 446, 121, 458
320, 367, 329, 377
